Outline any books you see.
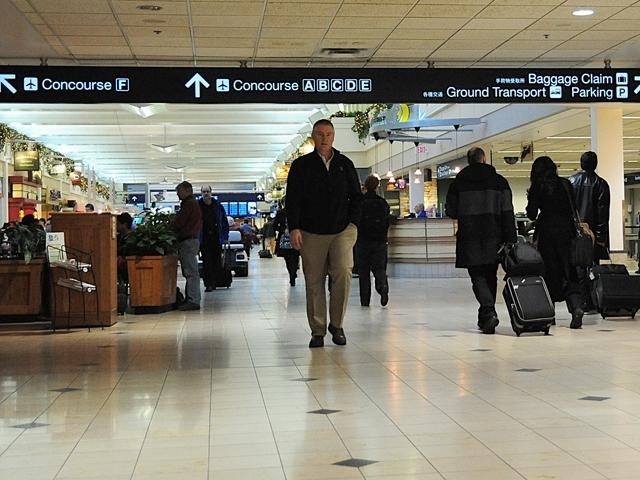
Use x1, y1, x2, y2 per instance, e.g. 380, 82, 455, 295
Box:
57, 278, 96, 292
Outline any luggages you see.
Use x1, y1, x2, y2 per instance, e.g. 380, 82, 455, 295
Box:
502, 234, 556, 336
215, 241, 233, 288
595, 272, 640, 319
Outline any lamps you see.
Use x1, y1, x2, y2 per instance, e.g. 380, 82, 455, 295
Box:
389, 140, 396, 183
414, 127, 422, 176
413, 142, 422, 184
148, 125, 180, 156
373, 133, 380, 181
122, 104, 163, 120
453, 125, 461, 174
386, 130, 393, 178
164, 154, 187, 173
276, 163, 290, 178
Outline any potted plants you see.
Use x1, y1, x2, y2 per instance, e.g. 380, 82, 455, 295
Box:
0, 221, 50, 322
119, 202, 180, 314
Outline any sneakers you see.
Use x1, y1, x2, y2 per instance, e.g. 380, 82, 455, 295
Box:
179, 301, 200, 311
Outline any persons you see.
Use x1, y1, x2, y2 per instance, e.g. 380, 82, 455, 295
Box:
413, 202, 429, 218
288, 119, 363, 347
167, 180, 203, 311
353, 173, 390, 307
198, 183, 229, 291
23, 198, 138, 281
445, 148, 518, 333
527, 156, 587, 329
227, 214, 276, 258
567, 150, 610, 313
274, 195, 302, 286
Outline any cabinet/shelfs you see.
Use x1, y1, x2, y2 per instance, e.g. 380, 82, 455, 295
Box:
48, 245, 104, 332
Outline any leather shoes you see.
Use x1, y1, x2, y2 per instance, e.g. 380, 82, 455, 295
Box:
328, 323, 346, 345
570, 308, 584, 328
380, 283, 388, 306
482, 315, 499, 334
308, 335, 324, 347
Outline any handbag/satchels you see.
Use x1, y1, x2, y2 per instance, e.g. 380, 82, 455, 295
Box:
279, 232, 293, 249
500, 240, 545, 276
570, 229, 594, 267
592, 264, 629, 289
258, 249, 272, 258
576, 208, 595, 244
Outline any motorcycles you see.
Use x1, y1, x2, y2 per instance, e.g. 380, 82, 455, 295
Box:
198, 229, 249, 278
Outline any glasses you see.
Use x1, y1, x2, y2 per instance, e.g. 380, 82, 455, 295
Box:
202, 190, 209, 193
